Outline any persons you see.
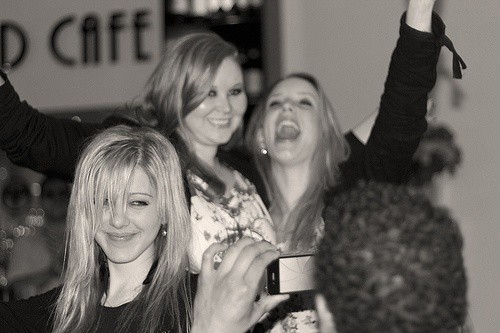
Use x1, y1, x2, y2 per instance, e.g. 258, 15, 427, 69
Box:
188, 180, 470, 333
0, 0, 436, 333
218, 0, 467, 264
0, 123, 261, 333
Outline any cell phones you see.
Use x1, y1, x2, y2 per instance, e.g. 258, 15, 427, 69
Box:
266, 253, 315, 295
